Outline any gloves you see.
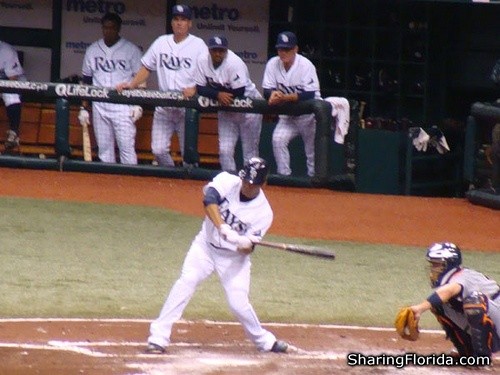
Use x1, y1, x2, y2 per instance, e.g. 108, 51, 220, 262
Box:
219, 224, 239, 246
78, 110, 90, 125
237, 237, 253, 255
130, 105, 143, 122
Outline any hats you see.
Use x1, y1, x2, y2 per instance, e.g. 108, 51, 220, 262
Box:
207, 37, 228, 49
172, 5, 191, 19
275, 32, 297, 48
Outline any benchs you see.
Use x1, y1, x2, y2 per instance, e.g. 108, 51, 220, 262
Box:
0, 102, 219, 165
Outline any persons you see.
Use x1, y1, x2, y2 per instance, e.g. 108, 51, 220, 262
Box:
146, 158, 287, 354
116, 3, 208, 166
78, 13, 146, 165
0, 39, 25, 149
196, 34, 266, 172
262, 30, 323, 175
395, 241, 500, 363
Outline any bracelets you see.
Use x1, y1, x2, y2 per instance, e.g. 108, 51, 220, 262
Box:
426, 292, 443, 311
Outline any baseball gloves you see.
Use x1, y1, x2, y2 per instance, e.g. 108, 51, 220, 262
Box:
394, 306, 419, 341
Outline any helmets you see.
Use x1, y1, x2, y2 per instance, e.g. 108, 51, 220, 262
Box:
425, 242, 462, 288
238, 157, 269, 185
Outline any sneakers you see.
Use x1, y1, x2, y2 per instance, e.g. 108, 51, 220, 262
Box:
4, 130, 19, 150
146, 343, 164, 354
270, 342, 290, 354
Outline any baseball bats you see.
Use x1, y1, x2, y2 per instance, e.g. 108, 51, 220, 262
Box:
82, 123, 92, 161
252, 241, 335, 260
